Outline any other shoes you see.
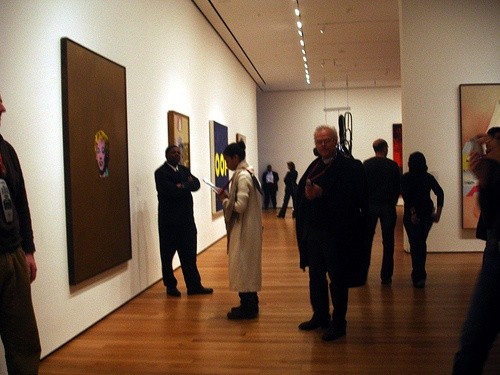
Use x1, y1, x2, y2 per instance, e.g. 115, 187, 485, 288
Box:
381, 278, 392, 287
412, 279, 426, 289
165, 286, 181, 297
321, 327, 347, 343
348, 281, 367, 288
187, 287, 213, 296
298, 320, 329, 331
277, 215, 284, 218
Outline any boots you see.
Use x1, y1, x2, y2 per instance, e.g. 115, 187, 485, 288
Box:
227, 295, 259, 320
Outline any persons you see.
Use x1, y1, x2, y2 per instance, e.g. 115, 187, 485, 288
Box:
95, 131, 110, 179
363, 139, 403, 286
401, 151, 444, 288
294, 126, 359, 344
451, 126, 500, 375
0, 97, 41, 375
262, 165, 279, 210
277, 162, 298, 218
154, 145, 213, 296
216, 142, 262, 319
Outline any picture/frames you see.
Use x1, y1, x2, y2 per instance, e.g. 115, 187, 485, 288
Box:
460, 82, 500, 230
167, 111, 190, 172
60, 37, 133, 294
236, 133, 246, 147
209, 120, 228, 215
